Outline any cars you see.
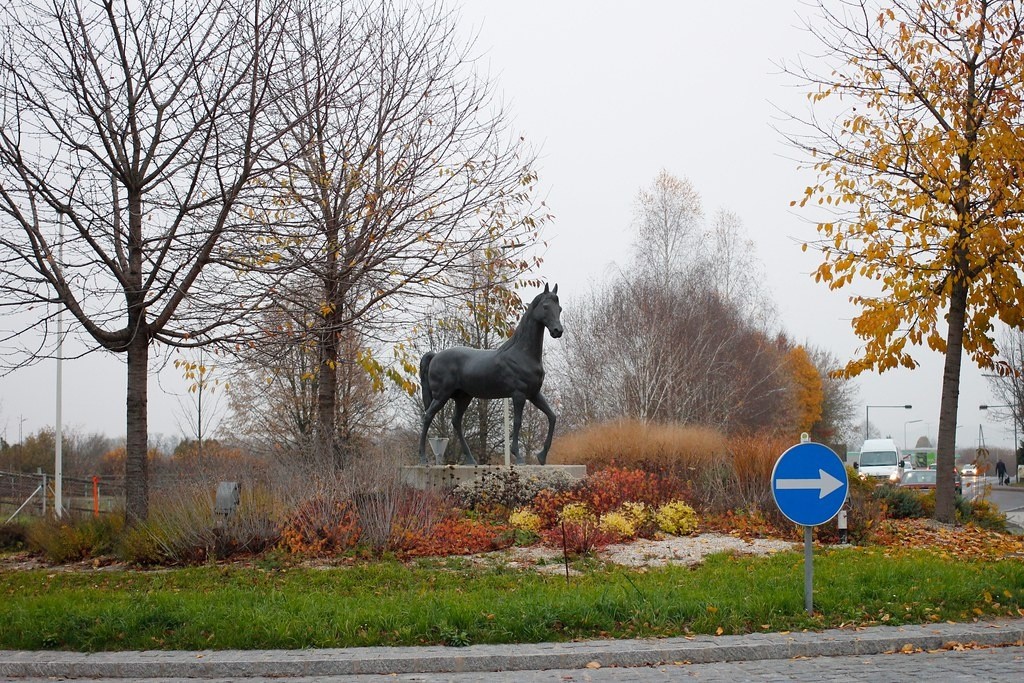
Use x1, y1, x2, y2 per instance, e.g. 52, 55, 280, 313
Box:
898, 471, 937, 496
962, 464, 978, 476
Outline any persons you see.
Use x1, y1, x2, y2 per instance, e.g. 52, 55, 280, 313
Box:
996, 459, 1006, 486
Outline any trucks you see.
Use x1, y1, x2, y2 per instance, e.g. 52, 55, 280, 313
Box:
847, 448, 938, 473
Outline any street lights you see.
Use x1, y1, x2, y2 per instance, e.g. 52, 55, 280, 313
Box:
904, 419, 922, 454
983, 375, 1018, 484
866, 405, 912, 439
502, 244, 528, 466
52, 206, 73, 521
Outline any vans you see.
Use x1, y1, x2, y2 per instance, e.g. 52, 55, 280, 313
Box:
928, 464, 963, 496
854, 438, 905, 486
903, 462, 915, 472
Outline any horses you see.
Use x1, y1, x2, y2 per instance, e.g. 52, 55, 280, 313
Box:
417, 282, 563, 466
